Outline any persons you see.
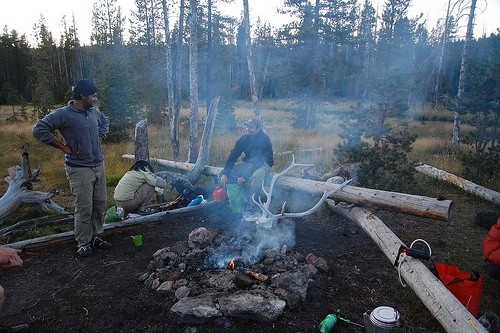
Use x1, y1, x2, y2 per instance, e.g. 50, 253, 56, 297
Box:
114, 161, 167, 214
0, 248, 24, 308
33, 79, 113, 255
219, 118, 273, 218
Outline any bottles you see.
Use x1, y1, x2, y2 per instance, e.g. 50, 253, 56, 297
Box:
319, 310, 340, 333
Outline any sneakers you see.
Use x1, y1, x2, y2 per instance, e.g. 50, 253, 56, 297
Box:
77, 244, 92, 257
90, 235, 112, 250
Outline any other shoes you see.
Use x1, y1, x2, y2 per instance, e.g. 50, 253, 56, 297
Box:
139, 208, 157, 214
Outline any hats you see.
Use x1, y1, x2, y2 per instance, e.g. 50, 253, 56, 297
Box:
244, 118, 262, 129
74, 80, 98, 95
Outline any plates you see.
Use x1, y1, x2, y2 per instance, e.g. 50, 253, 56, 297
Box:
370, 306, 400, 328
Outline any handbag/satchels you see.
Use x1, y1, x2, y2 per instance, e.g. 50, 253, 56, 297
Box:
431, 256, 483, 319
104, 206, 124, 223
224, 183, 251, 214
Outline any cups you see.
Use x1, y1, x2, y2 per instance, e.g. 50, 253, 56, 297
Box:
133, 235, 142, 246
